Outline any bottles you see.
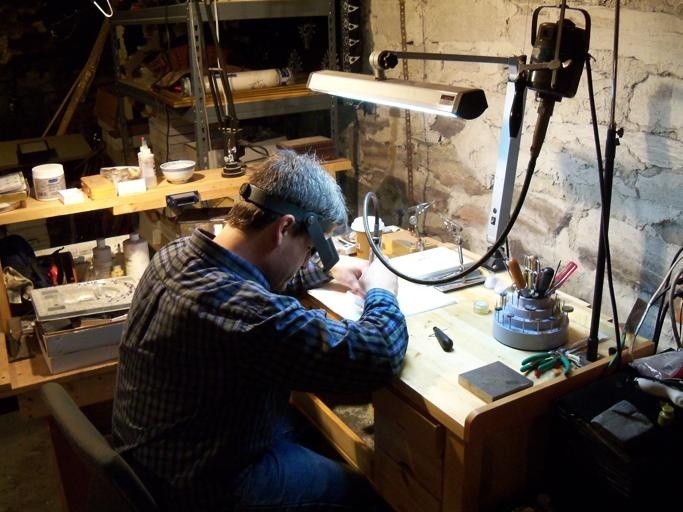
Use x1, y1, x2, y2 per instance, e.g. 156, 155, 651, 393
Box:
92, 231, 150, 278
32, 162, 67, 202
137, 144, 157, 188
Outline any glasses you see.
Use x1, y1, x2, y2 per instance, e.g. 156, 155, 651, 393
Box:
303, 212, 340, 274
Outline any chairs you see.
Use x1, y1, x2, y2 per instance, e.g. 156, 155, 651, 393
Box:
37, 379, 159, 511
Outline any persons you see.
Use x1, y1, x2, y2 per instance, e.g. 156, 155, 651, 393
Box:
112, 150, 409, 507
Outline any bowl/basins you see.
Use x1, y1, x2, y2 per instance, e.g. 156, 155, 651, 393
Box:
159, 159, 197, 184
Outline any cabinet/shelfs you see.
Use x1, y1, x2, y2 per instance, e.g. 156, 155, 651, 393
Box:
1, 157, 358, 421
101, 2, 343, 169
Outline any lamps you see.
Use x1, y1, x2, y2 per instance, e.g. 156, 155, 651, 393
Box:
305, 43, 532, 273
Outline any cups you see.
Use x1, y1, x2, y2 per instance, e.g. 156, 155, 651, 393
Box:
351, 215, 384, 261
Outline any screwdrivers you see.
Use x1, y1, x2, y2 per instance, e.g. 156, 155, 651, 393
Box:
503, 255, 578, 298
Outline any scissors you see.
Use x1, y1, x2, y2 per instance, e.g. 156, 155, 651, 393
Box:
520, 344, 588, 376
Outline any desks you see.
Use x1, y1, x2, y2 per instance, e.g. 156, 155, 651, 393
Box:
298, 223, 655, 509
